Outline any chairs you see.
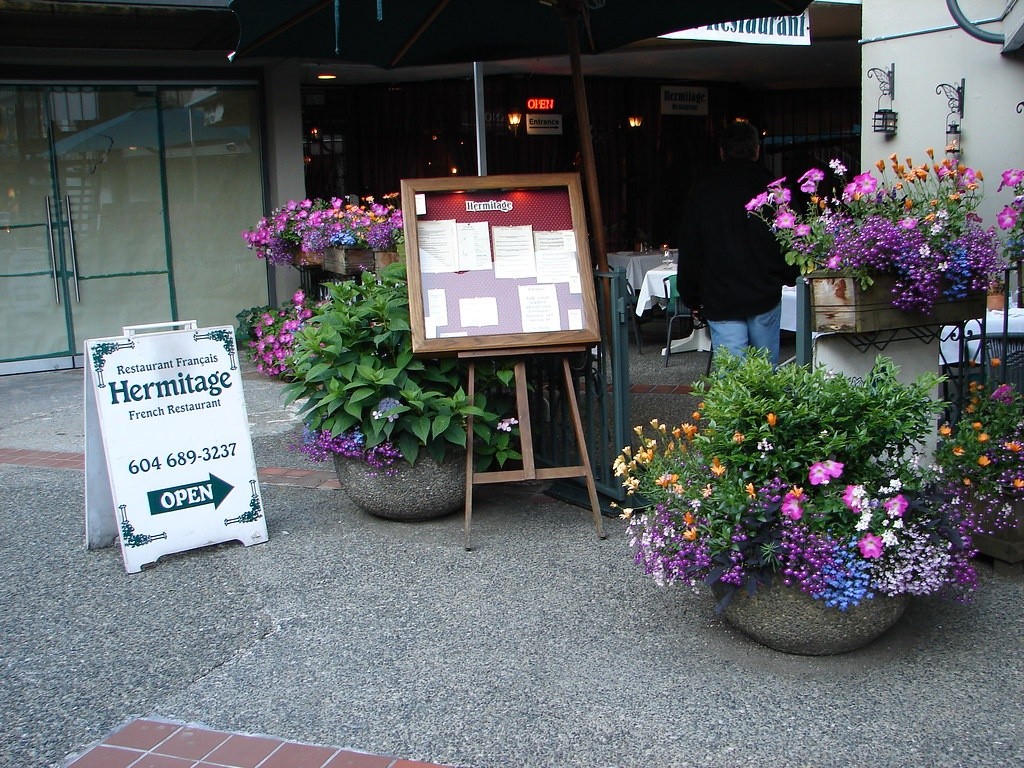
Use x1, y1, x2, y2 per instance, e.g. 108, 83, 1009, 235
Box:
608, 264, 642, 355
662, 274, 694, 367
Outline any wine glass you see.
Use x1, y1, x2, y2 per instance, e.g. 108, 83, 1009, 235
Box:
661, 251, 673, 269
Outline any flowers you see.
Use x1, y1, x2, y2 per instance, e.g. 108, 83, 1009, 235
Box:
996, 169, 1024, 269
746, 137, 1010, 317
987, 271, 1006, 295
610, 343, 987, 612
932, 354, 1024, 536
231, 192, 534, 474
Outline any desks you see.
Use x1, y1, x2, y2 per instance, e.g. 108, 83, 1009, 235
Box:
606, 249, 797, 366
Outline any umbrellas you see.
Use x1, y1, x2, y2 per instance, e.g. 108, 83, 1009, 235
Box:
231, 1, 817, 413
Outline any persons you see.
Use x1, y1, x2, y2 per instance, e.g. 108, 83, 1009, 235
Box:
677, 123, 783, 362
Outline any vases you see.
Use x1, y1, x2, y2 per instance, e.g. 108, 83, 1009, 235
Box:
332, 446, 477, 522
987, 294, 1004, 311
322, 245, 374, 277
973, 497, 1024, 564
709, 571, 914, 660
373, 247, 398, 270
295, 247, 324, 265
805, 272, 987, 335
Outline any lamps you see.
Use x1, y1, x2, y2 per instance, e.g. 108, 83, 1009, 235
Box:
627, 113, 642, 131
866, 63, 898, 136
935, 77, 965, 155
506, 109, 523, 138
